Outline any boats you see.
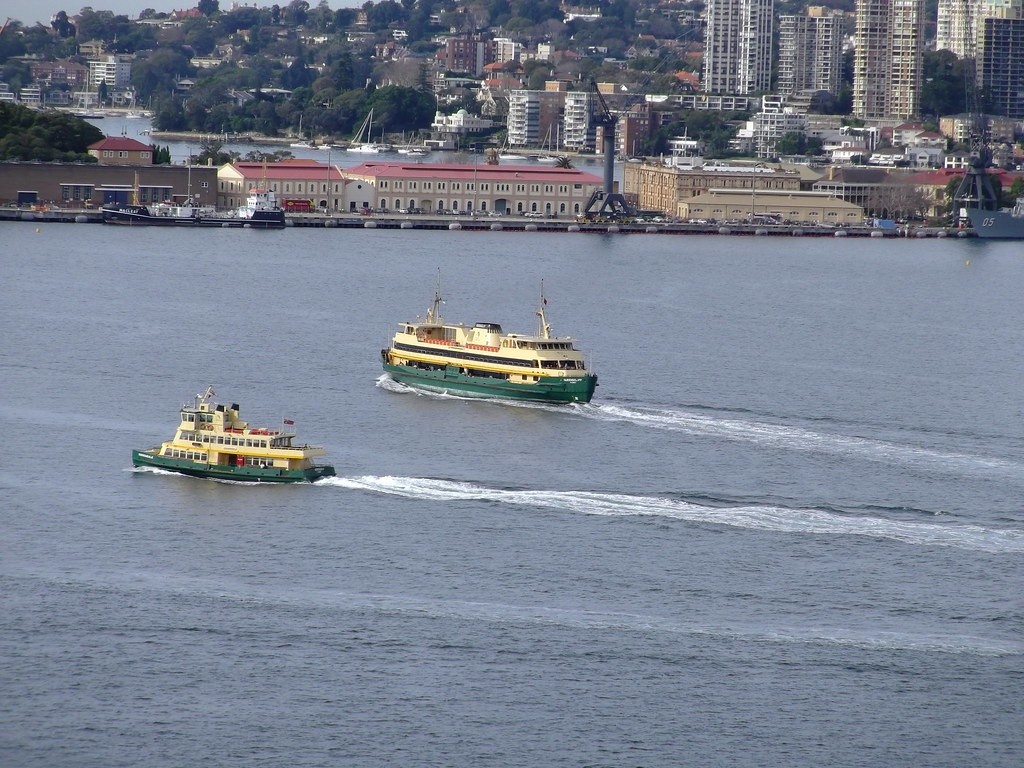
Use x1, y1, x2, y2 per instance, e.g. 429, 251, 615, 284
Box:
380, 266, 600, 406
102, 156, 286, 230
130, 385, 337, 486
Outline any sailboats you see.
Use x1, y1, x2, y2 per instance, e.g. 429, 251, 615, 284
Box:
497, 117, 564, 163
69, 71, 154, 120
290, 107, 429, 157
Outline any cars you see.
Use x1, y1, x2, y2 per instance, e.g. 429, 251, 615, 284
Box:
576, 212, 587, 218
436, 208, 460, 215
634, 216, 708, 224
520, 211, 544, 218
794, 219, 811, 226
397, 207, 427, 214
373, 207, 388, 213
473, 209, 503, 217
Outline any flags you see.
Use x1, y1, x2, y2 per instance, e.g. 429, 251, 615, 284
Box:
284, 419, 294, 425
